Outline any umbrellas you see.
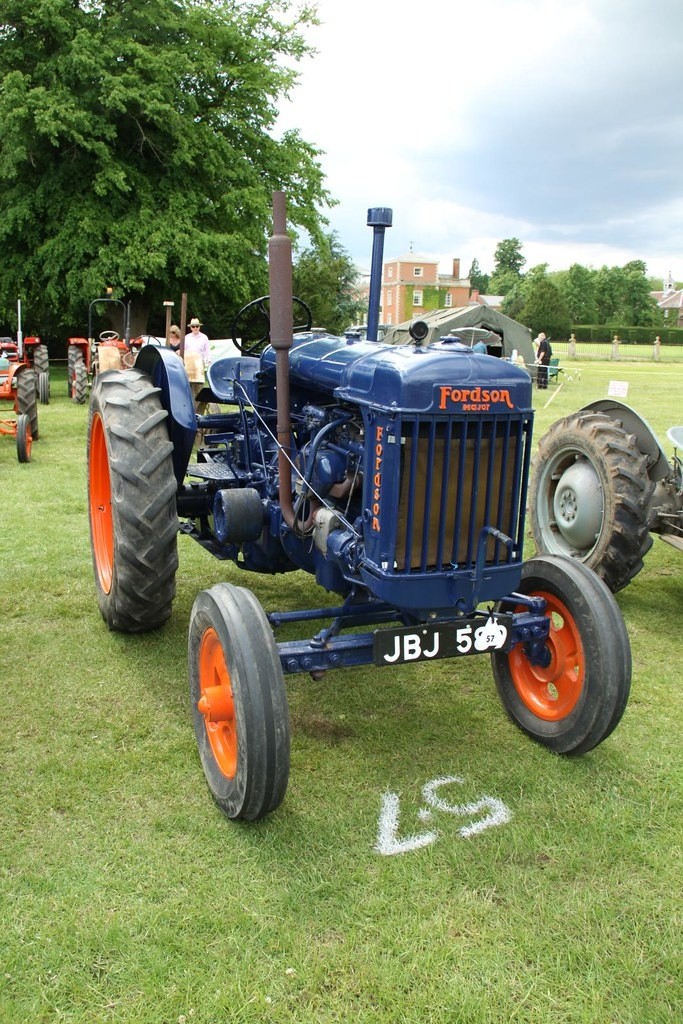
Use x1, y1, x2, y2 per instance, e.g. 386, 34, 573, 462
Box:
451, 326, 503, 349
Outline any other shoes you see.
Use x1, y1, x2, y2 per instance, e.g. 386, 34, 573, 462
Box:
543, 386, 547, 389
538, 386, 542, 389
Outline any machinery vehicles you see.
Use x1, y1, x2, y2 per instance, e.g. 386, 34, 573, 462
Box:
523, 397, 682, 602
85, 213, 633, 824
0, 348, 39, 464
68, 296, 143, 405
0, 299, 50, 405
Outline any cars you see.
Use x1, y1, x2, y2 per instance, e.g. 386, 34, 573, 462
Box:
343, 323, 395, 343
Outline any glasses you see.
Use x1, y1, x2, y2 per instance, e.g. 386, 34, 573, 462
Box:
169, 331, 176, 334
191, 325, 199, 328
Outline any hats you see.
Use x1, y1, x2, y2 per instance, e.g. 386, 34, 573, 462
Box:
187, 318, 203, 326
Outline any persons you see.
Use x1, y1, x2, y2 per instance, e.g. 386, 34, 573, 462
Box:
166, 325, 181, 352
185, 317, 211, 401
473, 340, 487, 355
533, 332, 552, 390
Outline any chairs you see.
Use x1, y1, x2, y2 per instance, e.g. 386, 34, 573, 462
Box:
549, 358, 559, 383
207, 356, 261, 402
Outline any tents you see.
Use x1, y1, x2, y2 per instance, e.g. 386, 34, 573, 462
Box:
378, 305, 538, 384
